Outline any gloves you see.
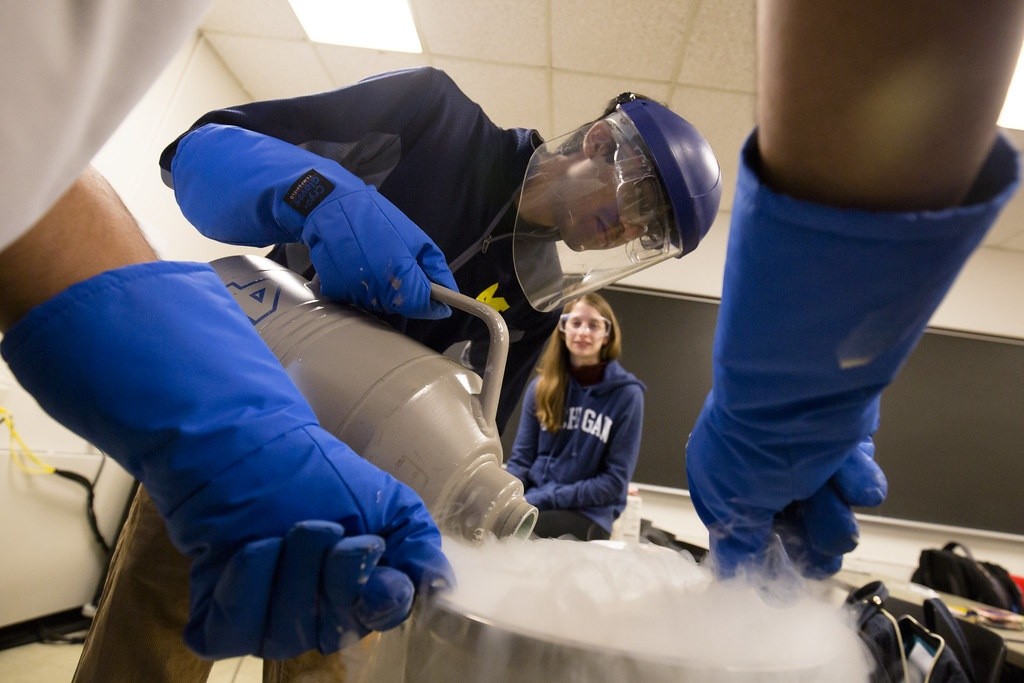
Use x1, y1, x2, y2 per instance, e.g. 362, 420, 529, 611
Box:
684, 128, 1021, 610
171, 124, 458, 320
0, 261, 450, 658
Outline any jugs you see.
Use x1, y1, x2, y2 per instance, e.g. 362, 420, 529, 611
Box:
211, 254, 539, 540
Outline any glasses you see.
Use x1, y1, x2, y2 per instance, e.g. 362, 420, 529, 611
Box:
558, 313, 612, 337
611, 123, 671, 261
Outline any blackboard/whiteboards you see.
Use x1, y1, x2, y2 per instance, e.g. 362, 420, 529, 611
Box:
501, 276, 1024, 542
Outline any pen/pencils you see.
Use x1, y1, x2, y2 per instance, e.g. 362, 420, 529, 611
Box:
1006, 638, 1024, 644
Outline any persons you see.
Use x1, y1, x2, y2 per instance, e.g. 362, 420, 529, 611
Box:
71, 63, 722, 682
502, 290, 646, 544
1, 0, 1024, 663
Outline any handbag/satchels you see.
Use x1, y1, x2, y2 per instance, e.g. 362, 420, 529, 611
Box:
852, 581, 977, 683
912, 542, 1019, 610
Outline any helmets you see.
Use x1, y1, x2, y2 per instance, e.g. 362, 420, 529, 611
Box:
621, 100, 722, 259
